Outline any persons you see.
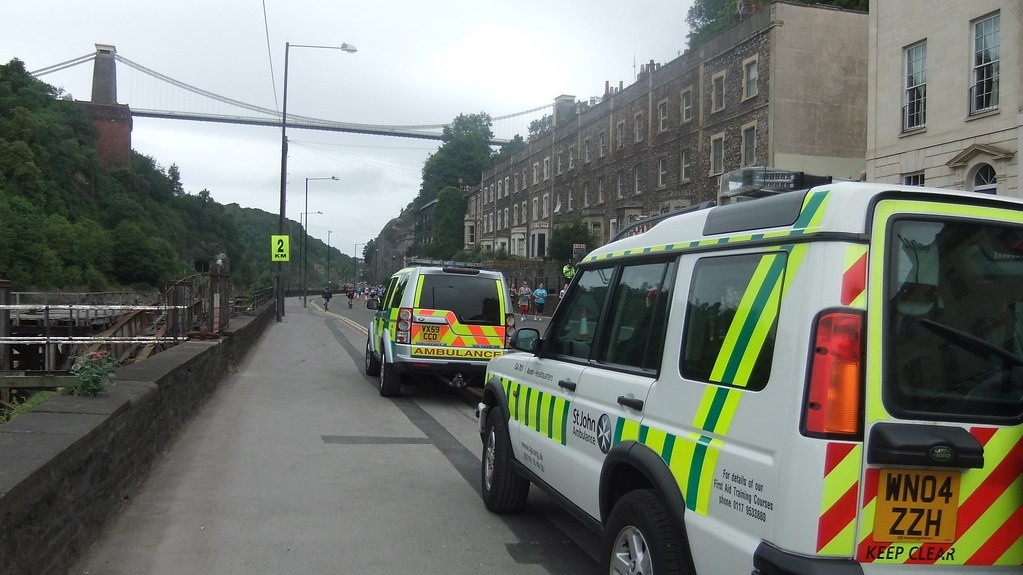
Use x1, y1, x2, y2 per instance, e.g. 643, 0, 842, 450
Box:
507, 283, 518, 306
322, 287, 332, 310
533, 283, 547, 321
559, 283, 568, 300
355, 282, 383, 302
517, 281, 532, 321
344, 285, 355, 306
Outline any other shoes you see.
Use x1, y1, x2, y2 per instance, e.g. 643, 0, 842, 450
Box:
534, 316, 537, 321
521, 315, 527, 321
538, 317, 542, 322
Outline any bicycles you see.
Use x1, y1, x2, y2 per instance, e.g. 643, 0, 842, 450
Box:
323, 296, 329, 311
346, 293, 353, 309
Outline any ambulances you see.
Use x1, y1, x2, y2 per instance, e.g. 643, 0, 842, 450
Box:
366, 267, 515, 397
475, 163, 1023, 575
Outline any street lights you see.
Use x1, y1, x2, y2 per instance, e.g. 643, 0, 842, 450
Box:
299, 212, 323, 298
354, 243, 365, 282
277, 42, 357, 319
327, 231, 332, 288
304, 176, 340, 306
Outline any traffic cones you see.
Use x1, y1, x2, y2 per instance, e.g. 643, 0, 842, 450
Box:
575, 306, 590, 339
